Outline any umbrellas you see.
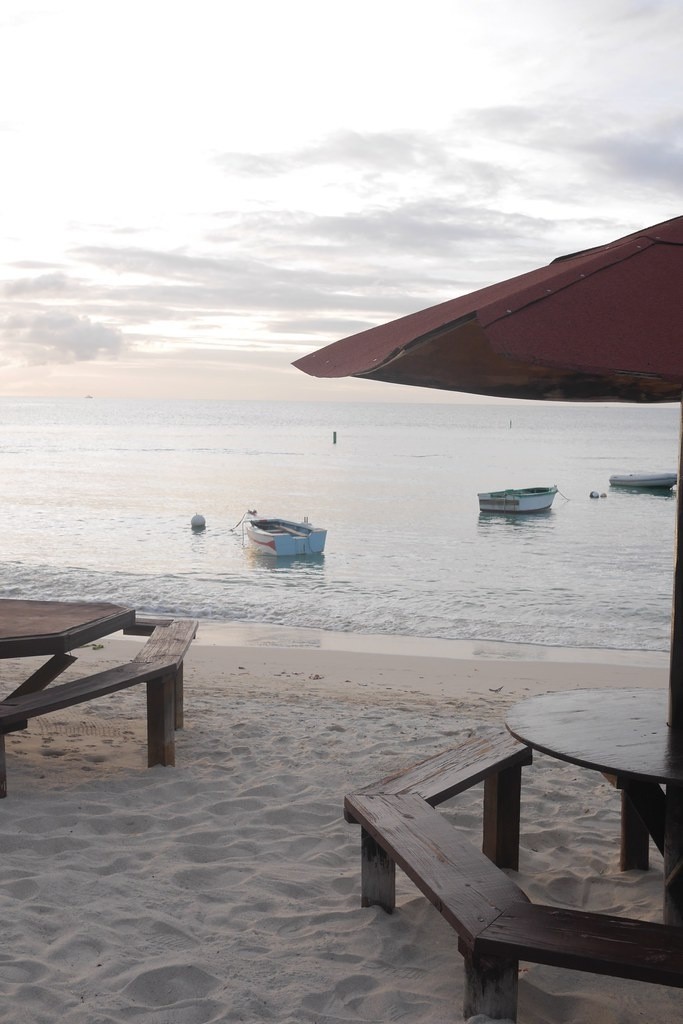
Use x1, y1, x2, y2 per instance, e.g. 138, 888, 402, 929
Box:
292, 216, 683, 728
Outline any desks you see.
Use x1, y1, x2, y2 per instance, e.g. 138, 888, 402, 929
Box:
503, 686, 683, 873
0, 598, 136, 733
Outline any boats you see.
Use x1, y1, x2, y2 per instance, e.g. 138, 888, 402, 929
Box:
243, 509, 328, 556
477, 484, 558, 513
608, 472, 677, 487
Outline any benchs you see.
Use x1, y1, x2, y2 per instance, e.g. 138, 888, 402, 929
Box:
341, 724, 683, 1024
264, 529, 285, 534
0, 618, 199, 798
